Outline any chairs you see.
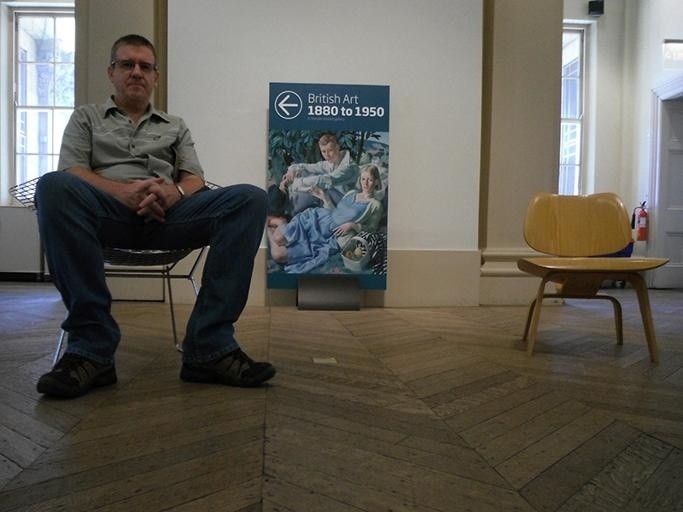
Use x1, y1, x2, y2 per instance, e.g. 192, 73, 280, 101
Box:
7, 167, 227, 369
515, 190, 670, 366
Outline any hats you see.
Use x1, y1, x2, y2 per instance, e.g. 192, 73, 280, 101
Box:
264, 184, 289, 216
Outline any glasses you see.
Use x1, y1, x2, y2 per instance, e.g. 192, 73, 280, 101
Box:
112, 59, 158, 71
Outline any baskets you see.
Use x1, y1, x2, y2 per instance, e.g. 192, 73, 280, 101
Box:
340, 230, 380, 271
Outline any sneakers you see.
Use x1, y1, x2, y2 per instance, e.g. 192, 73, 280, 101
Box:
37, 351, 118, 398
179, 348, 275, 387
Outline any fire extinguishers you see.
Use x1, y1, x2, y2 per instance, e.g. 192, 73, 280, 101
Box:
631, 201, 650, 242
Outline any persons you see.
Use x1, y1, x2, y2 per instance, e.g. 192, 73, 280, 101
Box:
268, 164, 385, 275
35, 35, 276, 399
278, 135, 361, 218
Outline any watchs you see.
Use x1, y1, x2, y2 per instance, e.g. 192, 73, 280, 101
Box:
172, 184, 185, 200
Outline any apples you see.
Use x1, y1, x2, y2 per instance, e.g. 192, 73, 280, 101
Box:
354, 247, 362, 257
346, 250, 352, 258
